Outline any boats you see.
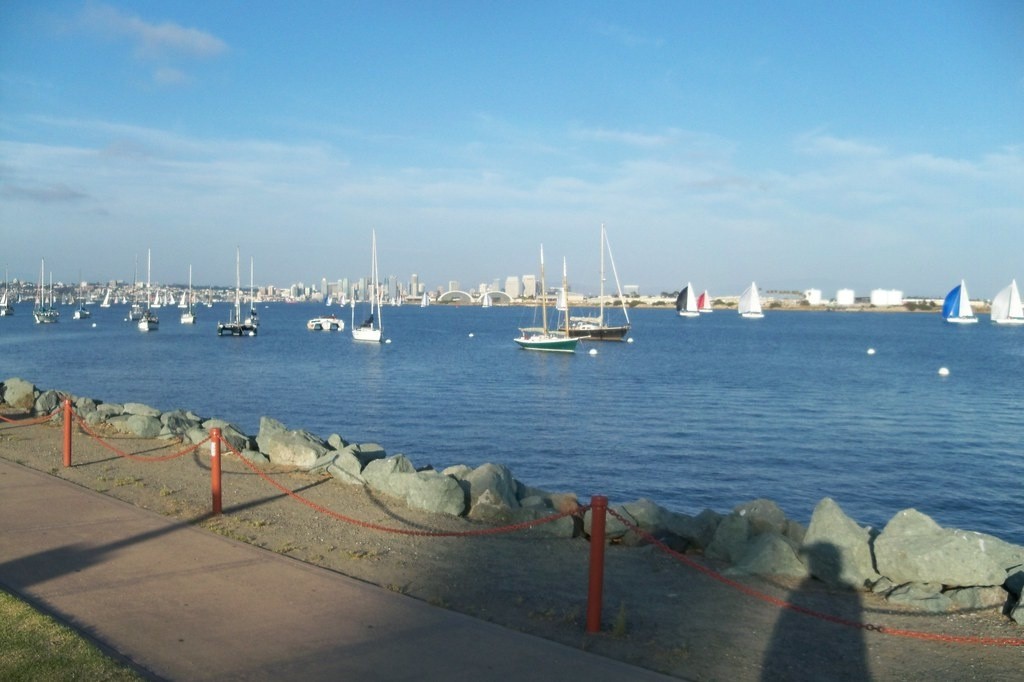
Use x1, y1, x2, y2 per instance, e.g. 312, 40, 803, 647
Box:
307, 318, 344, 331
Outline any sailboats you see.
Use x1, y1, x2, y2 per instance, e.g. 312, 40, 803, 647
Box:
349, 225, 383, 343
74, 274, 91, 319
128, 257, 143, 321
557, 223, 631, 342
419, 292, 430, 308
33, 257, 59, 324
674, 282, 699, 317
697, 289, 712, 313
326, 294, 356, 308
0, 263, 14, 317
181, 264, 196, 325
942, 281, 979, 323
217, 244, 257, 337
391, 295, 402, 307
11, 274, 214, 309
138, 248, 159, 330
990, 280, 1024, 324
735, 282, 764, 319
513, 242, 590, 353
245, 256, 260, 326
